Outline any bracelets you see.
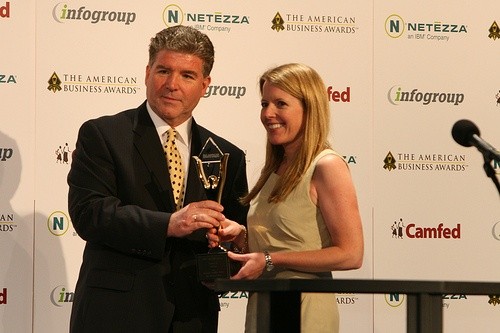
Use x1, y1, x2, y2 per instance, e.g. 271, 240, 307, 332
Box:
232, 224, 248, 254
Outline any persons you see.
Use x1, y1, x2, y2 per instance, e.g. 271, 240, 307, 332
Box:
211, 61, 365, 333
66, 24, 250, 333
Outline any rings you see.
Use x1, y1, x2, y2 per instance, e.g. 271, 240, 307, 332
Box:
204, 233, 209, 240
194, 215, 197, 222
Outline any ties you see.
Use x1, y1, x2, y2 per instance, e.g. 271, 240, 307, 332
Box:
164, 129, 185, 212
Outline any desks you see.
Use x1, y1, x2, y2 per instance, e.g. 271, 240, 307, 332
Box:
215, 279, 500, 333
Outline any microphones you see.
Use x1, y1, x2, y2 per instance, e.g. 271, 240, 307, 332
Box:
451, 119, 500, 162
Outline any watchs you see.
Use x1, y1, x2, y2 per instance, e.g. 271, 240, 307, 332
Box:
263, 249, 277, 275
